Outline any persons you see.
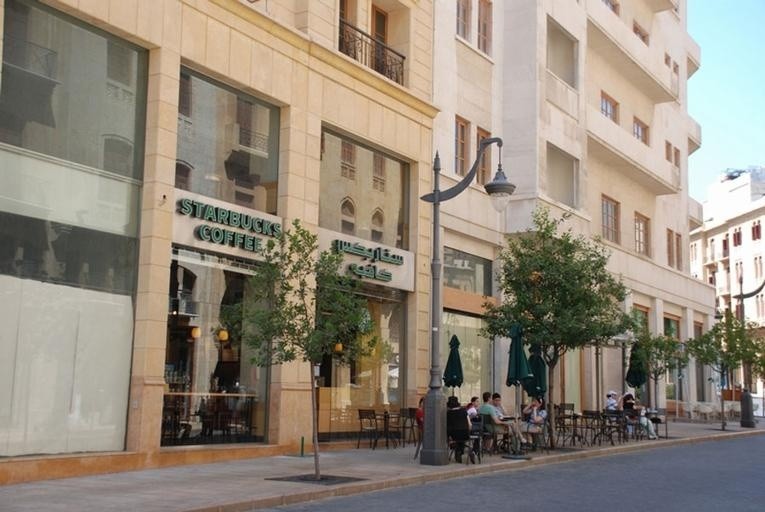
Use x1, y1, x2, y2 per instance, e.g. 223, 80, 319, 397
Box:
622, 393, 658, 439
416, 392, 548, 464
606, 390, 632, 434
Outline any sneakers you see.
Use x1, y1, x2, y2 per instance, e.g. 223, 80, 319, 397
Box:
469, 449, 476, 465
454, 449, 462, 462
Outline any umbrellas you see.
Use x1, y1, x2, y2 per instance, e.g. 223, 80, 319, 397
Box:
625, 342, 646, 388
443, 334, 463, 395
528, 344, 547, 395
506, 324, 535, 404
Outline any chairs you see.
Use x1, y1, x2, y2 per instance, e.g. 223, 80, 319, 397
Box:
447, 402, 663, 464
357, 405, 424, 459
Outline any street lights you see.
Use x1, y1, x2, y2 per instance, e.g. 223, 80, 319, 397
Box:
419, 135, 517, 467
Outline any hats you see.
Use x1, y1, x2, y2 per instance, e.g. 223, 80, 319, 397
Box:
607, 390, 616, 396
447, 396, 460, 408
